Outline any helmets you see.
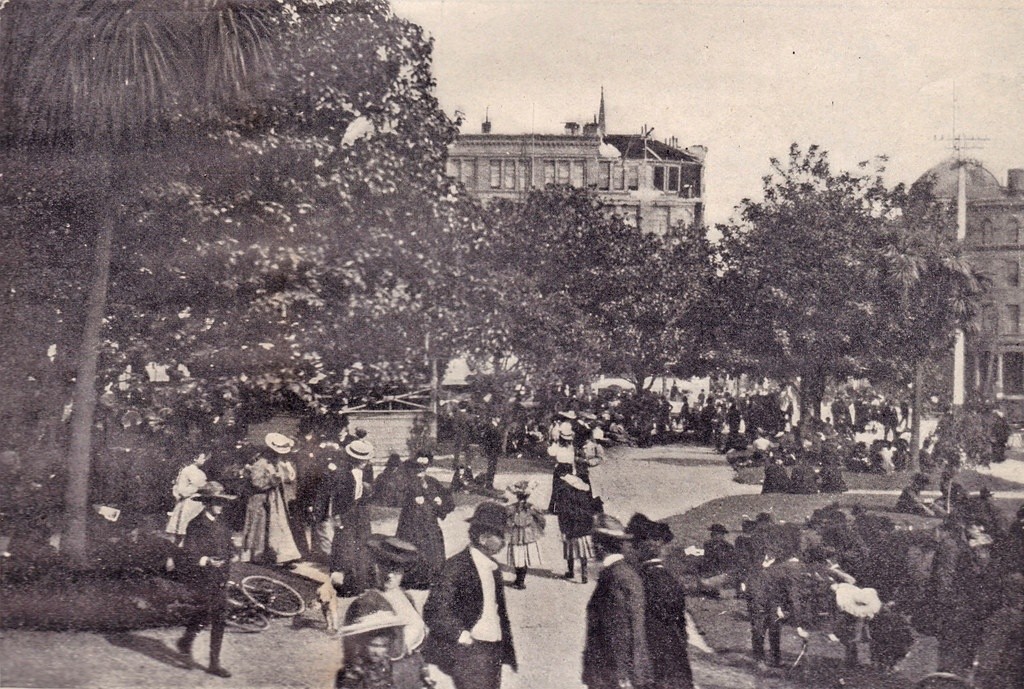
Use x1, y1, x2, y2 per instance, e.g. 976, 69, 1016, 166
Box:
332, 591, 411, 640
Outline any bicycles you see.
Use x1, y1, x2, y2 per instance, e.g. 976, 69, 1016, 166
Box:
224, 572, 307, 633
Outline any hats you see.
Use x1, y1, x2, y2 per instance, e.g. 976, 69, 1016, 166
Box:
466, 502, 516, 533
367, 536, 418, 565
559, 473, 590, 491
265, 432, 292, 454
560, 410, 576, 418
589, 514, 634, 539
624, 512, 674, 547
561, 424, 573, 440
706, 523, 729, 533
190, 483, 237, 500
344, 440, 374, 460
385, 454, 402, 468
507, 480, 536, 494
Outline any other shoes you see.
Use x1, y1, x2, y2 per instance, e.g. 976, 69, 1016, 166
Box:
177, 637, 195, 667
564, 571, 573, 578
508, 581, 526, 590
208, 662, 231, 676
582, 576, 588, 583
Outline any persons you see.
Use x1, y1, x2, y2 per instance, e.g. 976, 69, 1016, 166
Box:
920, 396, 1010, 475
419, 501, 519, 689
553, 473, 597, 583
450, 381, 679, 513
336, 538, 455, 689
166, 429, 455, 590
681, 388, 910, 494
506, 480, 547, 589
582, 473, 1024, 689
176, 481, 237, 678
